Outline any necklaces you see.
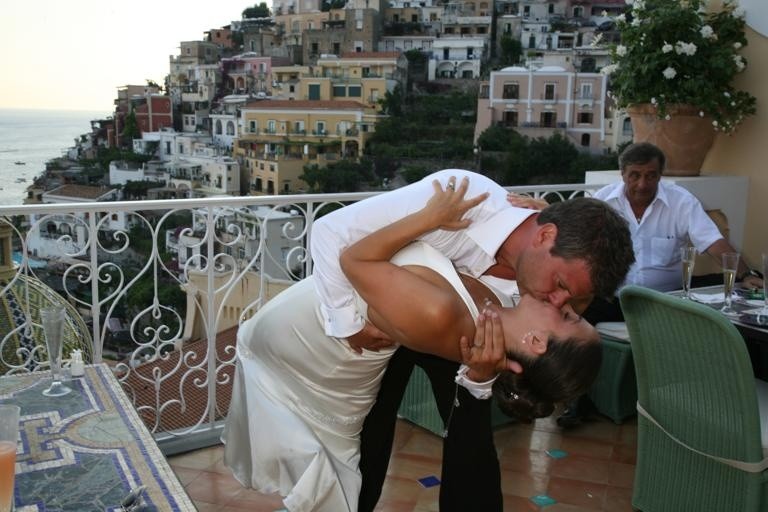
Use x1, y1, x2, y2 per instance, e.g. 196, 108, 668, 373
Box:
482, 297, 494, 315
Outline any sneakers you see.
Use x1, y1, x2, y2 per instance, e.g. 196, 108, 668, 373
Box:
555, 397, 596, 427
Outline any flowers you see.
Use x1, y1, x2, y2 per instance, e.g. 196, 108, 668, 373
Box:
587, 0, 758, 139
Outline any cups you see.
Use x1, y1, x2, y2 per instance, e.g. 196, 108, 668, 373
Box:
0, 404, 21, 512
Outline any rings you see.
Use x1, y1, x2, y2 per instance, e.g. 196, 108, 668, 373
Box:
446, 182, 454, 190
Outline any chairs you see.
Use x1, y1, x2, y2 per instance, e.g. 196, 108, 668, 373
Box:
618, 284, 768, 511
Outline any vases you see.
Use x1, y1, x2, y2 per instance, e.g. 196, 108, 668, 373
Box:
626, 104, 720, 177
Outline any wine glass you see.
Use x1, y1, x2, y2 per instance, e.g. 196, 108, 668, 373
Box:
38, 306, 73, 398
718, 253, 741, 316
679, 245, 699, 302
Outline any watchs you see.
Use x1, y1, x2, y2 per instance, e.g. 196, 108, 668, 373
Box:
741, 270, 763, 281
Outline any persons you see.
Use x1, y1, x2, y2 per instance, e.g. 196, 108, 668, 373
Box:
220, 175, 603, 512
556, 141, 765, 429
308, 168, 637, 512
